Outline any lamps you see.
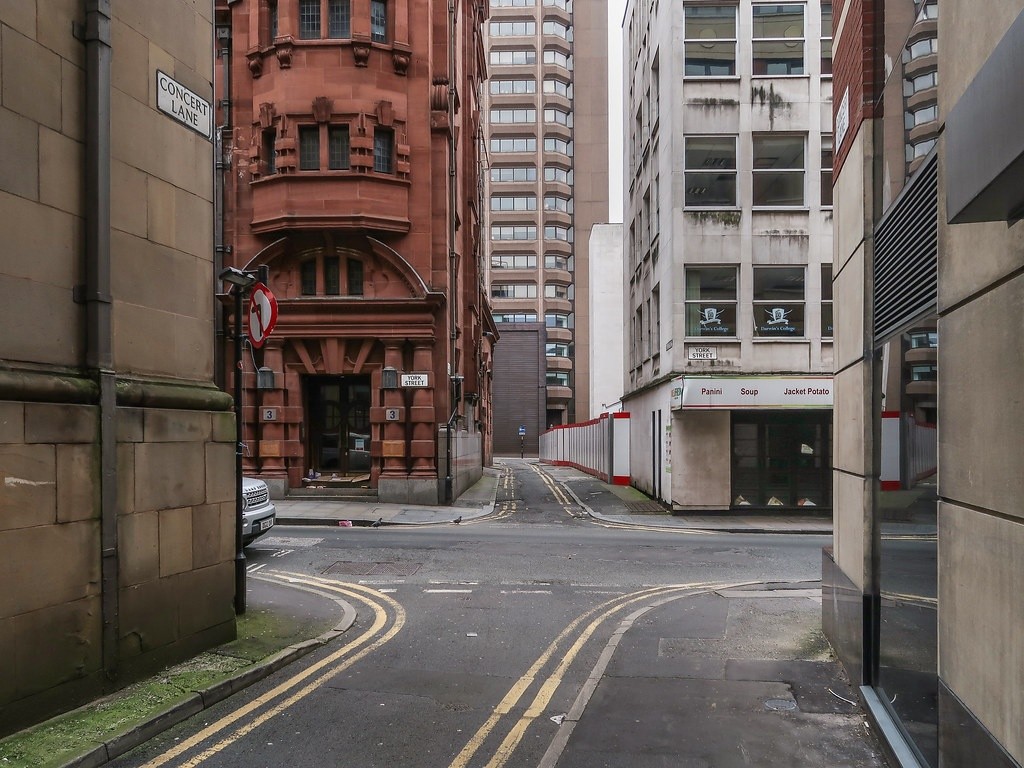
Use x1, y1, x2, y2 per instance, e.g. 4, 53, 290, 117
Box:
381, 366, 399, 389
257, 366, 274, 389
